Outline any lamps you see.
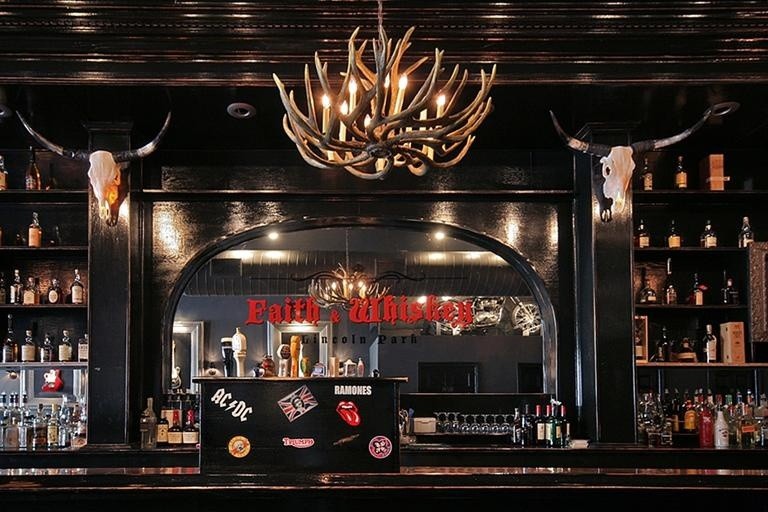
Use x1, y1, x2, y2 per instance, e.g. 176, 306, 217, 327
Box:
289, 230, 425, 312
273, 0, 496, 181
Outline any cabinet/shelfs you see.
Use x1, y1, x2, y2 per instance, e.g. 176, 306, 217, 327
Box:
631, 190, 768, 451
0, 190, 88, 455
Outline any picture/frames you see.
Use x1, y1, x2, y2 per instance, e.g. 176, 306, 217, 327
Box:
267, 321, 332, 375
173, 321, 203, 394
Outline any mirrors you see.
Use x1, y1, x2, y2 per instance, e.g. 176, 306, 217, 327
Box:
141, 190, 577, 424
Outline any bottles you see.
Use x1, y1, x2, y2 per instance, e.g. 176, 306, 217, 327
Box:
652, 320, 717, 364
15, 231, 26, 246
356, 358, 365, 377
637, 159, 655, 192
27, 211, 41, 249
0, 390, 86, 450
655, 384, 767, 448
24, 144, 41, 189
46, 162, 57, 189
0, 264, 85, 305
0, 157, 8, 189
2, 313, 87, 364
634, 216, 752, 249
49, 225, 62, 246
672, 154, 687, 188
511, 402, 571, 448
636, 255, 740, 307
137, 397, 200, 450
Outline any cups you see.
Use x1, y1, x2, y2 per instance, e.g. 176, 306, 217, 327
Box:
220, 337, 236, 376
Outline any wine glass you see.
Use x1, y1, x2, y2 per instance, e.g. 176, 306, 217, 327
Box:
636, 390, 667, 449
432, 410, 511, 433
